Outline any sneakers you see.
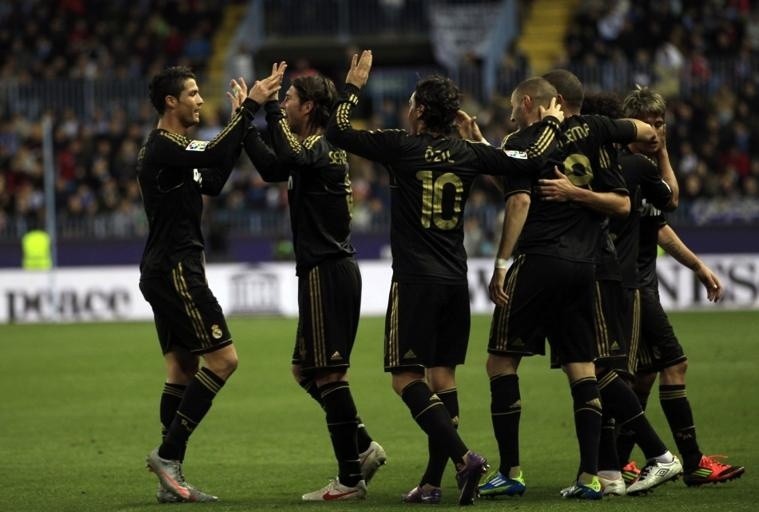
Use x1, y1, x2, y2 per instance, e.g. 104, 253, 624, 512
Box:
476, 467, 527, 497
682, 453, 746, 489
399, 481, 442, 503
155, 478, 218, 502
144, 444, 191, 501
358, 440, 389, 485
301, 474, 368, 501
559, 458, 640, 499
453, 450, 490, 506
625, 454, 684, 496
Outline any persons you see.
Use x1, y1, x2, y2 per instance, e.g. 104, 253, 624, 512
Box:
2, 99, 391, 222
216, 57, 393, 504
327, 44, 565, 502
136, 64, 284, 504
450, 1, 758, 498
0, 1, 232, 88
